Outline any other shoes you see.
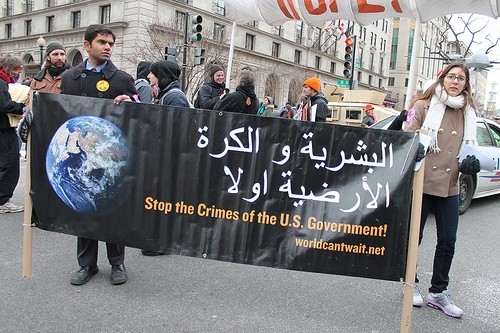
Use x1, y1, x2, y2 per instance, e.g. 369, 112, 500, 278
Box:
141, 249, 162, 256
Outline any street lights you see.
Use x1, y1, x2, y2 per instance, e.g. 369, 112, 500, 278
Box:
37, 35, 46, 72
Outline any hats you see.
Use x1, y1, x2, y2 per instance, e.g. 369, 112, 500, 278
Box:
266, 96, 274, 104
365, 105, 374, 111
304, 77, 320, 93
46, 41, 66, 55
210, 64, 223, 75
149, 62, 158, 78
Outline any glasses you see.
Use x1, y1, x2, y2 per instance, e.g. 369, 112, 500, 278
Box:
445, 74, 468, 82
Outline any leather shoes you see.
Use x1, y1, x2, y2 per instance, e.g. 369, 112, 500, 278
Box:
110, 263, 128, 284
70, 265, 98, 285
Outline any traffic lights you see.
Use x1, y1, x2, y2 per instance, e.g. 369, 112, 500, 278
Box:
343, 36, 354, 77
193, 48, 206, 65
164, 46, 177, 63
189, 15, 203, 44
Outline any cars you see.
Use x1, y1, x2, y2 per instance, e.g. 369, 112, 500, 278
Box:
334, 115, 500, 215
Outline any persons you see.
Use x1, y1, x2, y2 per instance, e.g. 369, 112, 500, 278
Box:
404, 60, 481, 318
282, 105, 295, 119
22, 41, 72, 117
61, 24, 141, 285
213, 69, 259, 114
141, 60, 195, 256
134, 61, 152, 102
280, 102, 291, 117
272, 104, 280, 116
361, 104, 377, 127
194, 64, 229, 110
0, 53, 27, 214
257, 96, 274, 116
293, 76, 329, 122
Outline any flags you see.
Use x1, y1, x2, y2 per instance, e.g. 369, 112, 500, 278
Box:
334, 21, 345, 39
324, 22, 333, 32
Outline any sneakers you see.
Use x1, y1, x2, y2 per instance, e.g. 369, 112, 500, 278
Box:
426, 290, 464, 318
402, 282, 424, 306
0, 198, 24, 213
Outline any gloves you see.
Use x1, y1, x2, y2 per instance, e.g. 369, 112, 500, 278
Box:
415, 142, 430, 162
459, 155, 480, 174
